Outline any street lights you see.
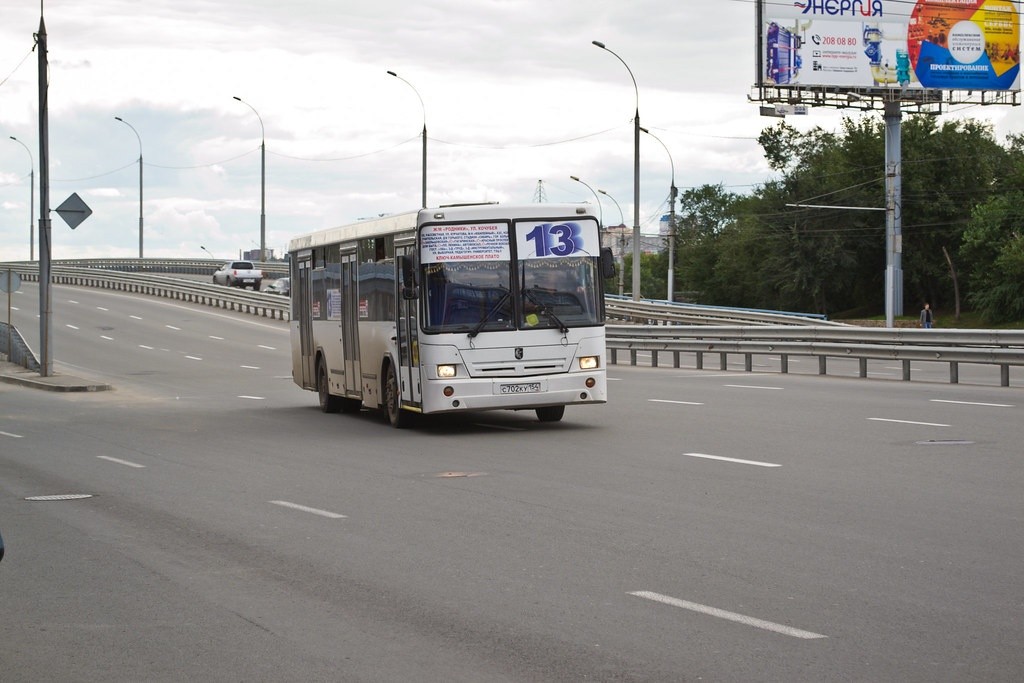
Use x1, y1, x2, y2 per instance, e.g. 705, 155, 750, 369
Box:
387, 70, 427, 209
10, 136, 34, 261
114, 116, 144, 259
597, 189, 625, 295
571, 175, 603, 255
233, 96, 266, 261
638, 127, 676, 301
594, 41, 641, 300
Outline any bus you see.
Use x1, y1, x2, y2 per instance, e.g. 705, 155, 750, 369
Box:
288, 201, 609, 428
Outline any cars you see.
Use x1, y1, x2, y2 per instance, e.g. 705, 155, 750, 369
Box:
263, 278, 290, 296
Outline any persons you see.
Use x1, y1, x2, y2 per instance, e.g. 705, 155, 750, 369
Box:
526, 271, 571, 312
919, 304, 934, 328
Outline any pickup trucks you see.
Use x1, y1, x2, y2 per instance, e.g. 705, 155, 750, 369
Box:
213, 260, 264, 291
